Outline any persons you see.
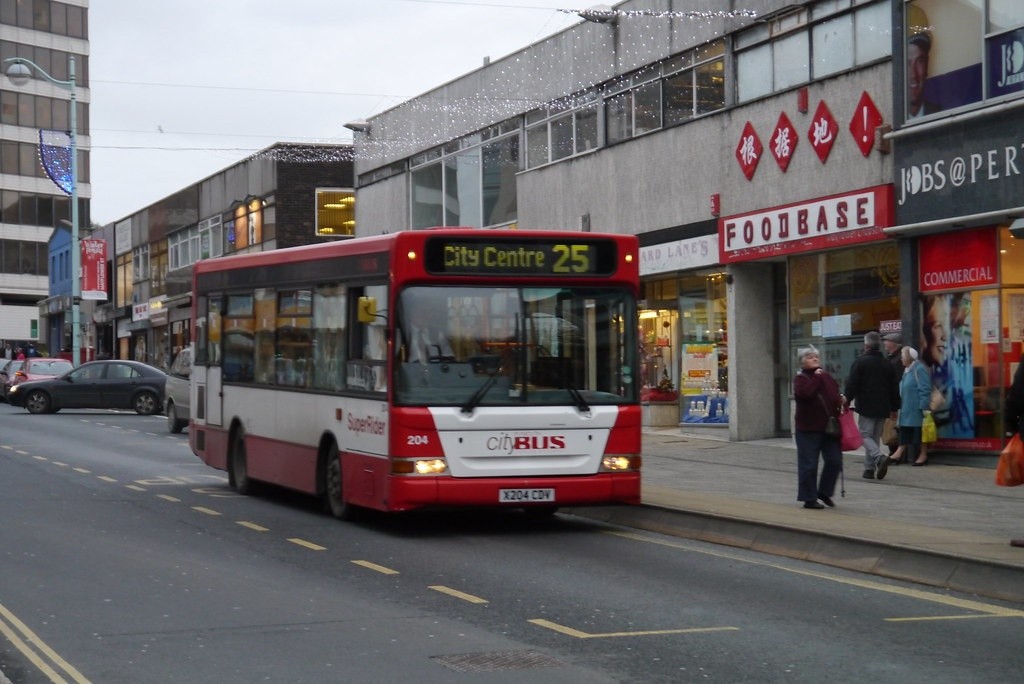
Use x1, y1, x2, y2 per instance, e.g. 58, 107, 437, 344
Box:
405, 301, 454, 363
793, 345, 847, 509
17, 348, 25, 360
922, 296, 946, 412
880, 333, 904, 375
888, 346, 932, 466
905, 5, 938, 119
1, 343, 16, 360
842, 331, 901, 478
1004, 352, 1024, 447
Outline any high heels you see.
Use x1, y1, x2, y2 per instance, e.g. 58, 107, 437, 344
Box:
913, 456, 928, 467
887, 457, 900, 465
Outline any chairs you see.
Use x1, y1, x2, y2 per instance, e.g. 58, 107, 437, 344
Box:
224, 325, 312, 385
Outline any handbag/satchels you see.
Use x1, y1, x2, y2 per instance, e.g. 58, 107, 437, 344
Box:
881, 418, 897, 444
837, 398, 863, 451
929, 386, 946, 411
827, 416, 841, 436
921, 412, 937, 442
996, 434, 1024, 486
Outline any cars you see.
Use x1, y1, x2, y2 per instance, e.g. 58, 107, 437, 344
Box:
161, 347, 191, 434
12, 358, 77, 387
6, 359, 169, 416
0, 360, 25, 400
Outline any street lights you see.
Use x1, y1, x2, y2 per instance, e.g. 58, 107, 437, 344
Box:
5, 55, 81, 370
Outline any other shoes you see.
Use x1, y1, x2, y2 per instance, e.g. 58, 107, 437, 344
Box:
804, 492, 835, 510
1011, 540, 1024, 547
863, 455, 890, 479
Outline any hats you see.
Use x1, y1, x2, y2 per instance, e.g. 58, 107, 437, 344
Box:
902, 346, 918, 362
881, 333, 900, 343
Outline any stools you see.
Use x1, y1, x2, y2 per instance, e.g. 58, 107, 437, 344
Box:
976, 410, 996, 438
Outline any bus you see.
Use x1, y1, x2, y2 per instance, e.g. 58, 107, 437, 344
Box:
188, 226, 643, 522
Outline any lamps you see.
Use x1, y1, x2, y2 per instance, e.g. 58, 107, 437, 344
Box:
576, 2, 620, 29
229, 200, 242, 209
1011, 218, 1024, 240
243, 194, 256, 205
343, 123, 371, 136
